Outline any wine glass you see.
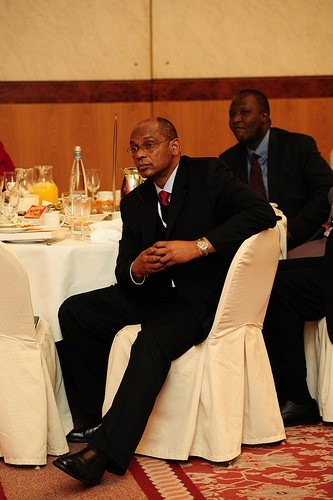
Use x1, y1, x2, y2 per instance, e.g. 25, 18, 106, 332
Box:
62, 193, 94, 241
0, 168, 33, 224
86, 168, 102, 200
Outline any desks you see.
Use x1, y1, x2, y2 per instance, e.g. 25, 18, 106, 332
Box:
0, 200, 286, 439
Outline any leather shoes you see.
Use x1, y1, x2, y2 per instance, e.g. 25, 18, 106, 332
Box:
66, 422, 104, 443
280, 398, 320, 427
52, 445, 107, 485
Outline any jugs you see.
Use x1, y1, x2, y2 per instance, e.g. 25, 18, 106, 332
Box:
120, 166, 146, 198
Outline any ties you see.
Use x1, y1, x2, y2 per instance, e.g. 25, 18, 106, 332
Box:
249, 153, 266, 201
159, 191, 170, 226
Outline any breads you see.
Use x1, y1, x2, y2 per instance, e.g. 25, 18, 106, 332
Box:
82, 200, 121, 208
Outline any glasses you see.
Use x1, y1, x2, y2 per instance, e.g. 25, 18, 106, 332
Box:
127, 137, 175, 157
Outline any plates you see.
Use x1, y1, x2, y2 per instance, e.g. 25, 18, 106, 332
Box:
1, 224, 53, 243
92, 214, 107, 221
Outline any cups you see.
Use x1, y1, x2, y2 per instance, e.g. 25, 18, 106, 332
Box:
94, 190, 122, 201
44, 212, 65, 226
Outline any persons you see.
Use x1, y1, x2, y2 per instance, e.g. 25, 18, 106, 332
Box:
218, 89, 333, 252
54, 117, 278, 485
258, 230, 333, 426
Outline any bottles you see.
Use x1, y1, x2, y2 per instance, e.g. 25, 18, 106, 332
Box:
70, 145, 87, 195
33, 165, 60, 207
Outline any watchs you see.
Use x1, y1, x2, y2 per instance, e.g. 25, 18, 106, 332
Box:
197, 237, 209, 255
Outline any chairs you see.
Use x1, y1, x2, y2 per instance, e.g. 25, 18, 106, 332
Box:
0, 226, 333, 468
